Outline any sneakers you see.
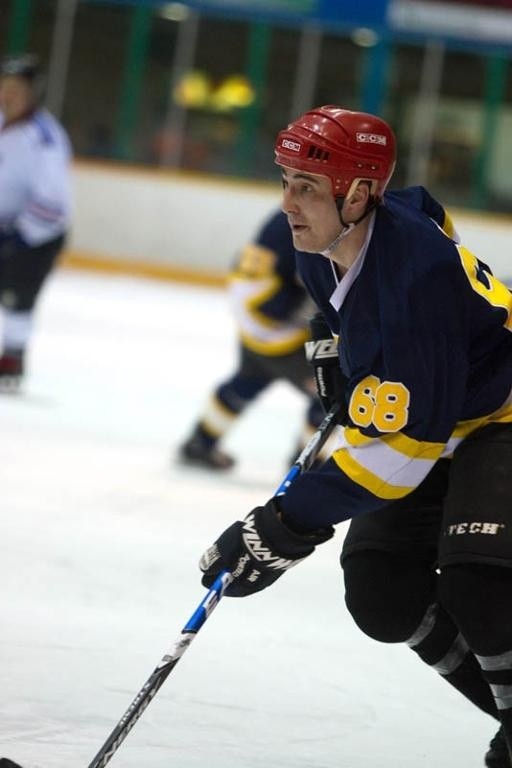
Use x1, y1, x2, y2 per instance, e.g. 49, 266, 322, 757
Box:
486, 718, 510, 767
183, 434, 234, 469
1, 354, 22, 376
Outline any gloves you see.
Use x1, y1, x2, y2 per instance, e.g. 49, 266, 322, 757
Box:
305, 315, 347, 425
200, 494, 335, 597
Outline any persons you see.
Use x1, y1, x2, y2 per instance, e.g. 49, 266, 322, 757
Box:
1, 53, 77, 387
167, 184, 327, 474
197, 100, 511, 768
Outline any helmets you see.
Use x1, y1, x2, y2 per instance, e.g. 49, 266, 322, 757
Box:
277, 105, 396, 199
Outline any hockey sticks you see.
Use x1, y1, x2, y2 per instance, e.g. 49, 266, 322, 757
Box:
1, 399, 346, 768
